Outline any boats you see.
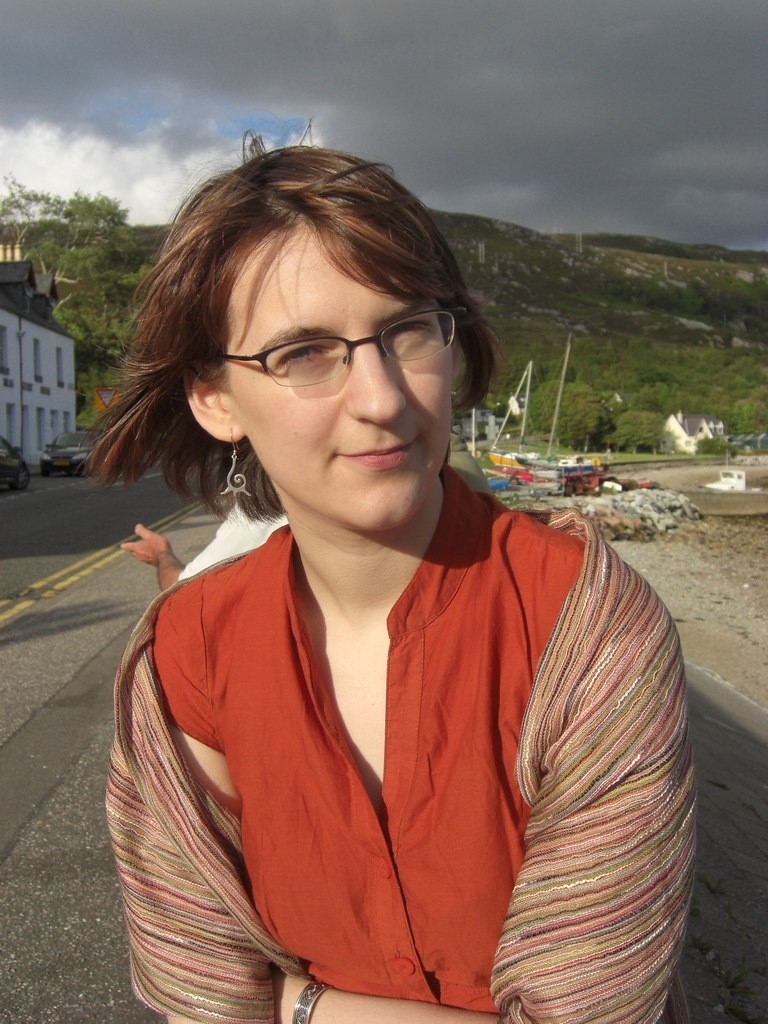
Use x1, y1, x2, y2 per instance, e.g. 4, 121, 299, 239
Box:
704, 469, 764, 492
480, 331, 657, 500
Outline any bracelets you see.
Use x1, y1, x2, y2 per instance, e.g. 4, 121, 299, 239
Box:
292, 981, 329, 1024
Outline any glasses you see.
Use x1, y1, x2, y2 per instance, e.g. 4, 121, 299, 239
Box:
211, 308, 472, 383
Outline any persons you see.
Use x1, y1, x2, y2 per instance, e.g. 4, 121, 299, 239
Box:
81, 120, 701, 1024
121, 502, 290, 592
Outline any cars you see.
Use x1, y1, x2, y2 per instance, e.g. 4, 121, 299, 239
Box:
0, 434, 31, 492
40, 429, 98, 477
